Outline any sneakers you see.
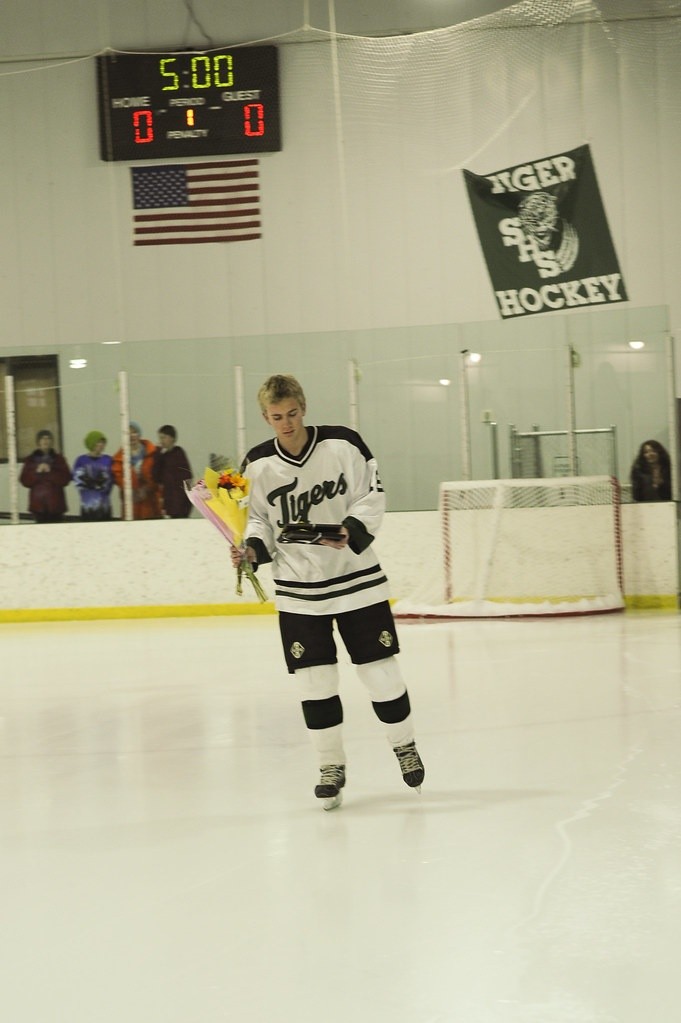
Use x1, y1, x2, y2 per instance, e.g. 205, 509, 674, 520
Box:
394, 741, 425, 794
314, 765, 346, 811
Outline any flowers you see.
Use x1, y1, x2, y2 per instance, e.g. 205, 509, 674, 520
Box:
182, 453, 269, 605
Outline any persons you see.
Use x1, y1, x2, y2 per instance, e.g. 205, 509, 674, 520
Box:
111, 421, 164, 521
628, 440, 672, 502
17, 430, 75, 521
229, 374, 426, 810
152, 425, 193, 519
69, 432, 116, 521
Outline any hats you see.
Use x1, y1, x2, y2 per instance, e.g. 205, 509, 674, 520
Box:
36, 429, 54, 446
130, 421, 142, 438
159, 425, 176, 438
85, 432, 107, 452
